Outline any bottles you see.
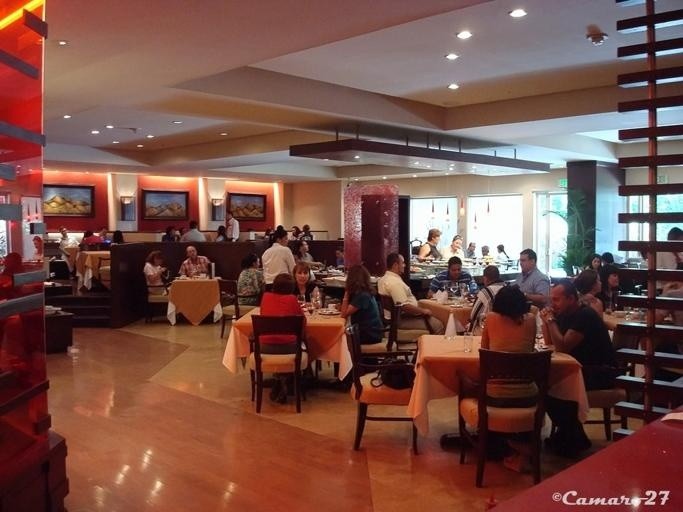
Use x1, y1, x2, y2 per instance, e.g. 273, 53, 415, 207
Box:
443, 311, 458, 340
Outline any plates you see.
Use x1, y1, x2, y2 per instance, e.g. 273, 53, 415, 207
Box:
314, 263, 378, 284
320, 309, 340, 316
411, 255, 472, 272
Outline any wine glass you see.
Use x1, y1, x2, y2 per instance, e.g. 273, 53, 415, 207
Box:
472, 253, 490, 271
431, 281, 477, 308
622, 285, 659, 322
297, 292, 322, 320
536, 324, 545, 349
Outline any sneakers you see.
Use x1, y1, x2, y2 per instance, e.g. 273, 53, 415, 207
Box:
503, 455, 525, 473
268, 381, 288, 405
544, 427, 592, 452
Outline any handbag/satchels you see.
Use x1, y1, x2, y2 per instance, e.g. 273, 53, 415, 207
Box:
380, 356, 415, 390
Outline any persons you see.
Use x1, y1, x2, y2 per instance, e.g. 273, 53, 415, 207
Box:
161, 210, 241, 243
325, 262, 384, 394
376, 252, 445, 336
538, 278, 619, 458
255, 272, 307, 406
247, 224, 344, 295
58, 226, 80, 282
177, 245, 212, 277
28, 235, 43, 262
410, 229, 509, 266
78, 230, 102, 245
426, 256, 478, 297
507, 248, 550, 312
111, 229, 123, 243
97, 226, 111, 246
235, 253, 264, 305
291, 261, 323, 383
478, 284, 538, 474
0, 251, 26, 347
465, 265, 507, 335
142, 249, 170, 294
570, 226, 683, 409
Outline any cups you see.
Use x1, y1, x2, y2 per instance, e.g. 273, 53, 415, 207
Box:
463, 331, 472, 351
327, 303, 337, 311
177, 272, 209, 281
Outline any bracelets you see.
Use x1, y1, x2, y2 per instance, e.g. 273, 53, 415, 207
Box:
523, 291, 526, 297
342, 298, 349, 301
545, 317, 557, 324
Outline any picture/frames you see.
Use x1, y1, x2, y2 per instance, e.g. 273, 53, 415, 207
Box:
229, 192, 268, 223
142, 188, 191, 220
42, 184, 97, 217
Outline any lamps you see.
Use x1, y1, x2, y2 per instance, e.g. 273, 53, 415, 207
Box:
428, 164, 495, 223
212, 199, 225, 221
121, 196, 136, 221
